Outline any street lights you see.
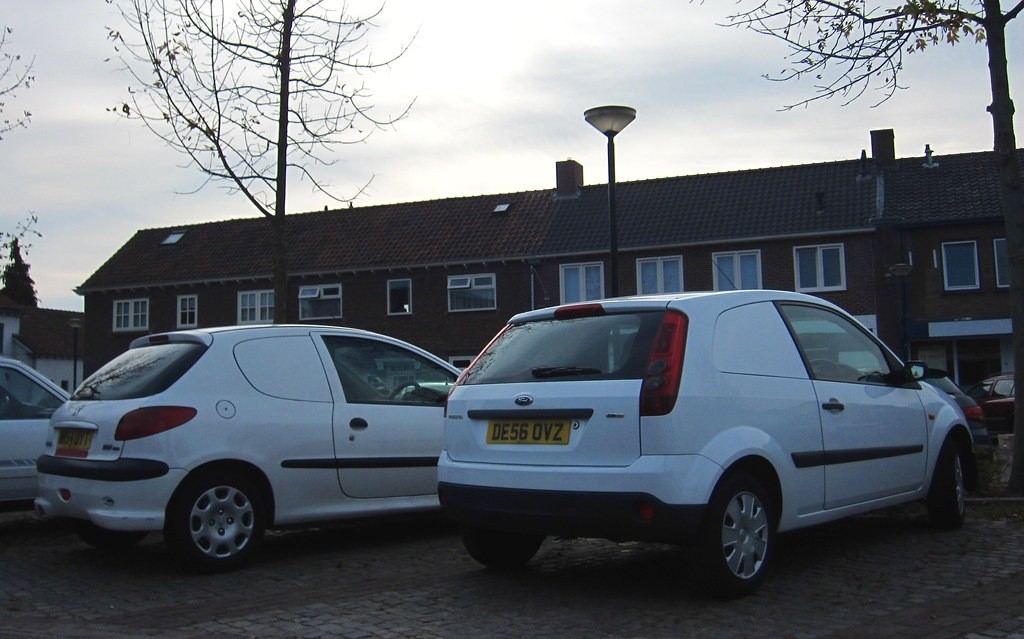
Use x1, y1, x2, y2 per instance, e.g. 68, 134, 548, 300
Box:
583, 106, 636, 369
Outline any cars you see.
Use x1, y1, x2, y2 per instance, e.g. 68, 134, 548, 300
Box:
401, 382, 454, 397
0, 358, 72, 513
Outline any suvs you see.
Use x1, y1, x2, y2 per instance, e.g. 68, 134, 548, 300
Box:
962, 371, 1015, 434
924, 367, 988, 491
33, 323, 464, 573
438, 289, 975, 601
